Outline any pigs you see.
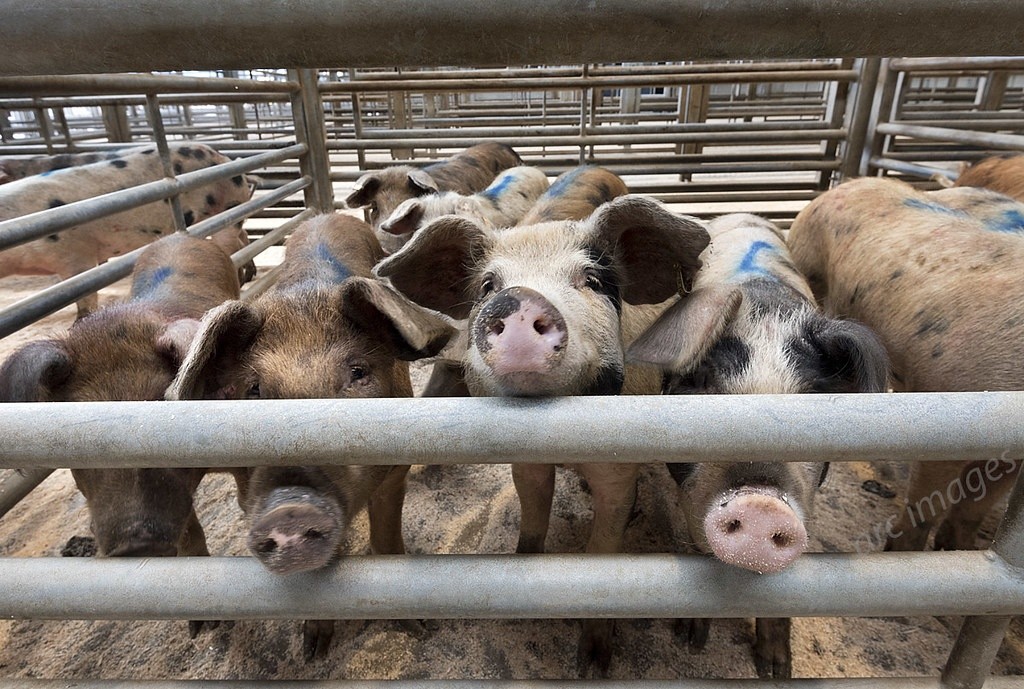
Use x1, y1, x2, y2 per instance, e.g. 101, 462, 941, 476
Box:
0, 141, 1024, 681
